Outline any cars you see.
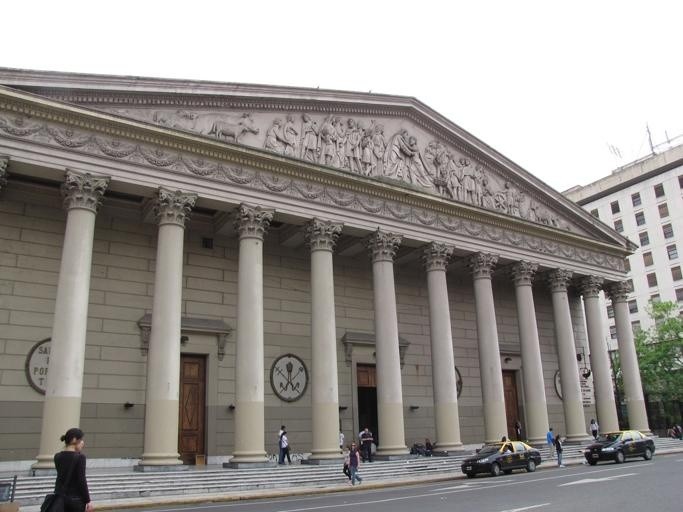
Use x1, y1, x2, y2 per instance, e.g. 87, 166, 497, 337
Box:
583, 429, 656, 466
463, 441, 541, 478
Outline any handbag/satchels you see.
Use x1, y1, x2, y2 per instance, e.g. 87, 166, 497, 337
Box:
41, 494, 65, 512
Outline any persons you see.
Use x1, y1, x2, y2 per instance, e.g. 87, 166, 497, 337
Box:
274, 415, 683, 489
264, 111, 516, 225
41, 428, 93, 512
